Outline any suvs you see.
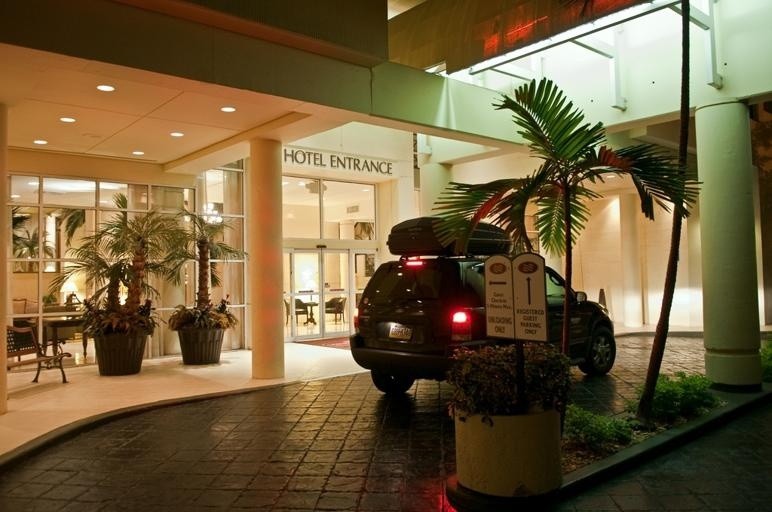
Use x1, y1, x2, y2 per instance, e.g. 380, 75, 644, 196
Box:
349, 217, 616, 393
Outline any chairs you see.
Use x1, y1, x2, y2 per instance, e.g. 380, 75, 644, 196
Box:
6, 324, 71, 384
325, 297, 347, 325
284, 299, 308, 325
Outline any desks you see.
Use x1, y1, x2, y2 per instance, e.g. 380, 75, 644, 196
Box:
10, 311, 98, 366
302, 302, 318, 325
40, 317, 87, 360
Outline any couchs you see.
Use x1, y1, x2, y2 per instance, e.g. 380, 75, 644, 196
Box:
11, 298, 75, 340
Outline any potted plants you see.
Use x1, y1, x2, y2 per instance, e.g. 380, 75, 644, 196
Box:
447, 342, 573, 498
44, 194, 179, 375
163, 205, 247, 364
